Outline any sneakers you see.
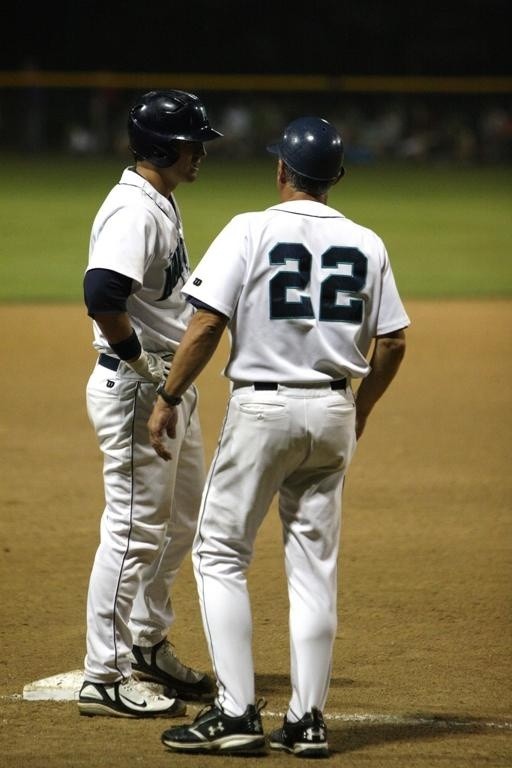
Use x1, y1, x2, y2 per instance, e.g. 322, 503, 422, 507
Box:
160, 697, 271, 756
270, 706, 329, 755
77, 675, 186, 718
131, 636, 218, 698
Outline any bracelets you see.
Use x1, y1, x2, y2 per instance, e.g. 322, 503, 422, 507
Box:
107, 328, 143, 361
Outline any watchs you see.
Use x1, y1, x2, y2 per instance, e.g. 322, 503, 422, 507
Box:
155, 379, 183, 405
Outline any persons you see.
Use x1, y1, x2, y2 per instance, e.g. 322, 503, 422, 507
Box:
78, 91, 223, 717
146, 118, 411, 768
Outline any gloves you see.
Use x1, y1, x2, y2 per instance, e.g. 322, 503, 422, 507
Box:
129, 348, 175, 383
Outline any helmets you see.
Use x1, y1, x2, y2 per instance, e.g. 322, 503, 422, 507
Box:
128, 90, 224, 166
267, 116, 345, 182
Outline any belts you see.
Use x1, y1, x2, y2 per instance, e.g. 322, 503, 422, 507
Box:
254, 378, 347, 390
97, 353, 121, 371
